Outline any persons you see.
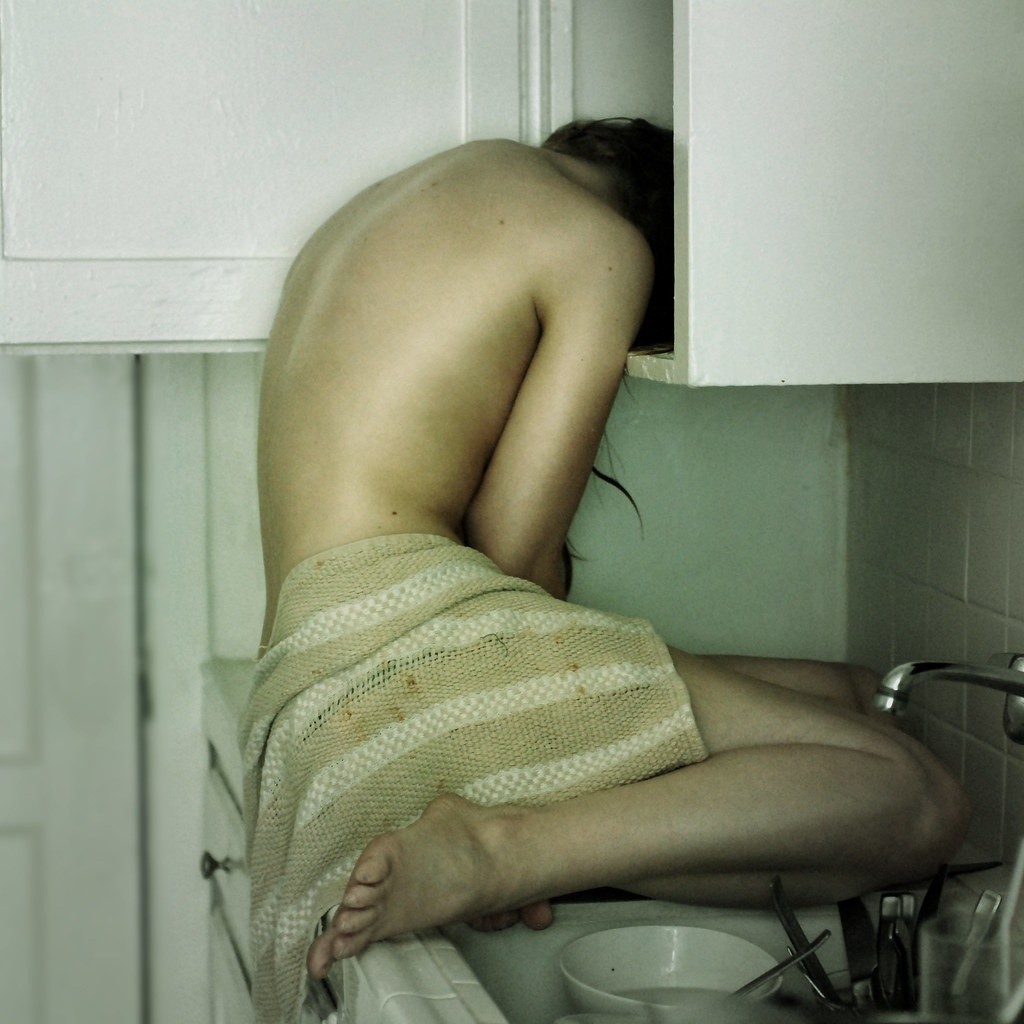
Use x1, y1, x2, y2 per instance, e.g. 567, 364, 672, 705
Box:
243, 119, 969, 1024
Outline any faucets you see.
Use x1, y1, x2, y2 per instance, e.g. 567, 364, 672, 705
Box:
872, 659, 1024, 744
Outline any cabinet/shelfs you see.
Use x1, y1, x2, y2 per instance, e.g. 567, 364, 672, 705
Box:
203, 739, 355, 1024
0, 0, 1024, 387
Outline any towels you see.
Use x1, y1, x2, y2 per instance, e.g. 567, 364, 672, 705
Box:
238, 535, 709, 1022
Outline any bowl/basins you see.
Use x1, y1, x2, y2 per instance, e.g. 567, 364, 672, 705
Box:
559, 925, 784, 1024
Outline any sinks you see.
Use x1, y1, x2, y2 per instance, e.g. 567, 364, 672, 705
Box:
431, 901, 1003, 1024
